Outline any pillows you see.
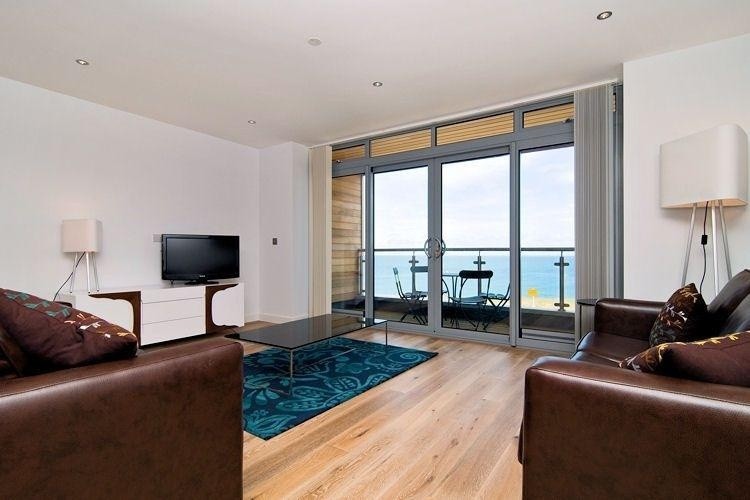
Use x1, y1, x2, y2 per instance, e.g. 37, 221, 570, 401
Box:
620, 330, 750, 387
650, 282, 708, 343
0, 288, 138, 367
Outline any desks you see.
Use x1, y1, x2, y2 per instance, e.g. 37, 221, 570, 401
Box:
442, 273, 476, 329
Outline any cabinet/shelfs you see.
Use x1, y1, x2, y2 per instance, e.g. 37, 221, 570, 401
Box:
60, 281, 245, 348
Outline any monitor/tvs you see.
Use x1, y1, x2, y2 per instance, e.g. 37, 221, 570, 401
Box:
159, 235, 243, 284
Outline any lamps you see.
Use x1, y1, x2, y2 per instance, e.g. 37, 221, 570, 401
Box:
62, 219, 101, 293
660, 123, 748, 307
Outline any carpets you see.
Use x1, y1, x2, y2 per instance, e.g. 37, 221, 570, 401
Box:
242, 337, 439, 440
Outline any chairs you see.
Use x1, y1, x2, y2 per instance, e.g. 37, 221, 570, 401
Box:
410, 266, 429, 292
480, 284, 511, 330
393, 267, 427, 325
449, 270, 493, 333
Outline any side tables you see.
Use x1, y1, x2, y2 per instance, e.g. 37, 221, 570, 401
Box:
577, 299, 597, 340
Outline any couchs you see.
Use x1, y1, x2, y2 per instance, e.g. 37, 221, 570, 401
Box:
517, 269, 750, 500
0, 301, 243, 500
